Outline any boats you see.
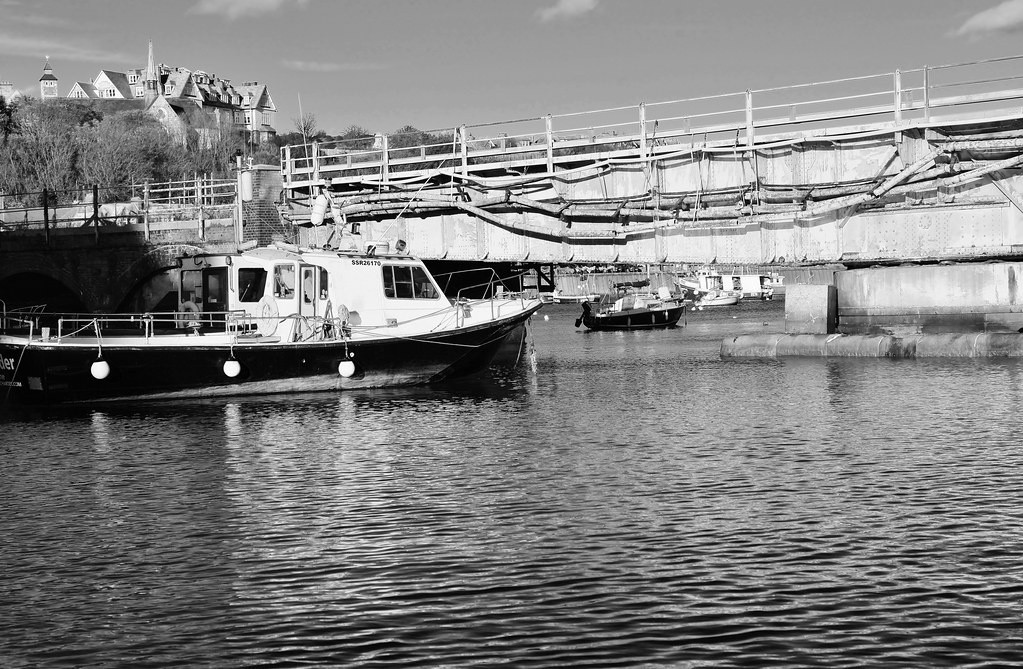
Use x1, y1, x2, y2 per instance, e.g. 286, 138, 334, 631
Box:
581, 266, 684, 332
696, 285, 738, 307
0, 151, 545, 409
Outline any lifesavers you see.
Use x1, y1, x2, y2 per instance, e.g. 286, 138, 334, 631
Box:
256, 295, 278, 337
177, 301, 199, 329
